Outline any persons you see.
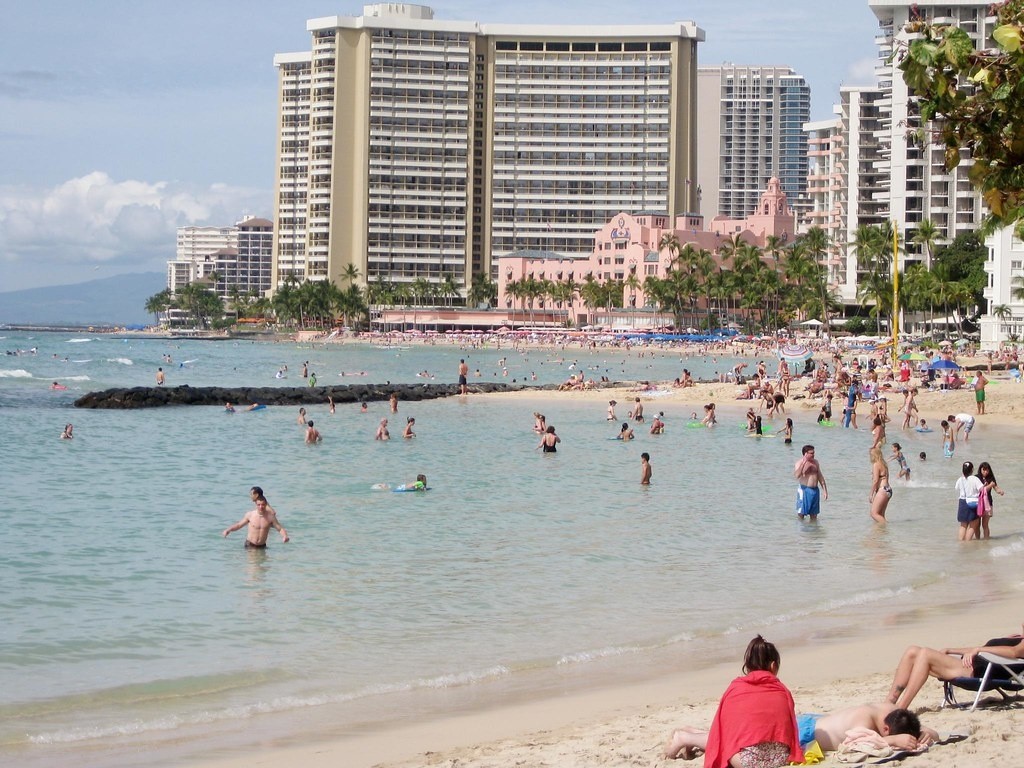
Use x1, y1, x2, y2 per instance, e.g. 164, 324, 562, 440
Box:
948, 413, 975, 440
304, 420, 322, 443
886, 621, 1024, 709
868, 446, 892, 522
974, 370, 989, 414
702, 634, 806, 768
459, 358, 469, 394
955, 461, 996, 541
794, 444, 828, 521
249, 486, 276, 527
667, 701, 940, 761
0, 329, 1024, 493
974, 462, 1004, 540
222, 497, 289, 549
885, 444, 911, 481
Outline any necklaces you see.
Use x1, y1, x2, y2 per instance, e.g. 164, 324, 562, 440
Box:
257, 510, 266, 517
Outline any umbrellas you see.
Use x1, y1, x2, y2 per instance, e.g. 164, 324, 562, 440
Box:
777, 345, 814, 376
112, 315, 979, 346
898, 351, 927, 374
928, 359, 960, 382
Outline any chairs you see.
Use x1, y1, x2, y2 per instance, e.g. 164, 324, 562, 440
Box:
938, 650, 1024, 712
749, 368, 912, 406
